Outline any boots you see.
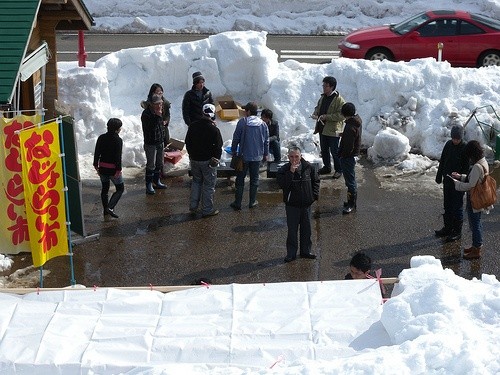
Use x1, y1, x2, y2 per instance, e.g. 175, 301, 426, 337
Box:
463, 243, 483, 258
342, 192, 357, 213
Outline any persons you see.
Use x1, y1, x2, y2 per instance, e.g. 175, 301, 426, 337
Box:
183, 71, 216, 127
312, 77, 346, 178
336, 103, 362, 214
261, 109, 281, 163
436, 125, 468, 241
141, 83, 170, 193
277, 146, 320, 262
432, 20, 444, 35
93, 118, 124, 218
451, 141, 489, 259
185, 103, 223, 216
345, 252, 373, 280
232, 102, 270, 210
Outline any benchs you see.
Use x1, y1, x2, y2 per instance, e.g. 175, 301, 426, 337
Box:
267, 161, 320, 178
188, 165, 236, 180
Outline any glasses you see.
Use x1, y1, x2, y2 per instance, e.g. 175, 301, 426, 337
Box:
321, 83, 330, 87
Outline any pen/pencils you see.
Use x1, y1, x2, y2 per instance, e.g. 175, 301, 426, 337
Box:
318, 117, 320, 122
452, 170, 460, 177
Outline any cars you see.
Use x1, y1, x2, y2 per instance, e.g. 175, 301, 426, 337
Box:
336, 7, 500, 69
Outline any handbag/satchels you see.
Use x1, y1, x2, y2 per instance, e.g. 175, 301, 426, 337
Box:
470, 175, 496, 211
230, 155, 243, 173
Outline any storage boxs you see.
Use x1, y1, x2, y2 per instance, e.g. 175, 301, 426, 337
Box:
239, 104, 263, 117
215, 95, 243, 122
164, 138, 186, 164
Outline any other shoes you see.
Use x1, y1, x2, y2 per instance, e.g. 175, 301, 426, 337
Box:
249, 200, 259, 208
434, 227, 448, 237
300, 252, 317, 259
103, 208, 108, 215
319, 166, 331, 174
447, 233, 461, 240
106, 208, 119, 218
333, 171, 342, 179
202, 209, 219, 216
230, 202, 241, 211
284, 255, 296, 262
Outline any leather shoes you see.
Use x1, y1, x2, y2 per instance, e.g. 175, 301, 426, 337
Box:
155, 178, 167, 188
146, 182, 155, 193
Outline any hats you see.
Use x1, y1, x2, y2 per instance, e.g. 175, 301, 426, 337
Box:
203, 104, 216, 117
241, 102, 257, 110
451, 125, 464, 139
152, 93, 164, 104
192, 71, 205, 84
